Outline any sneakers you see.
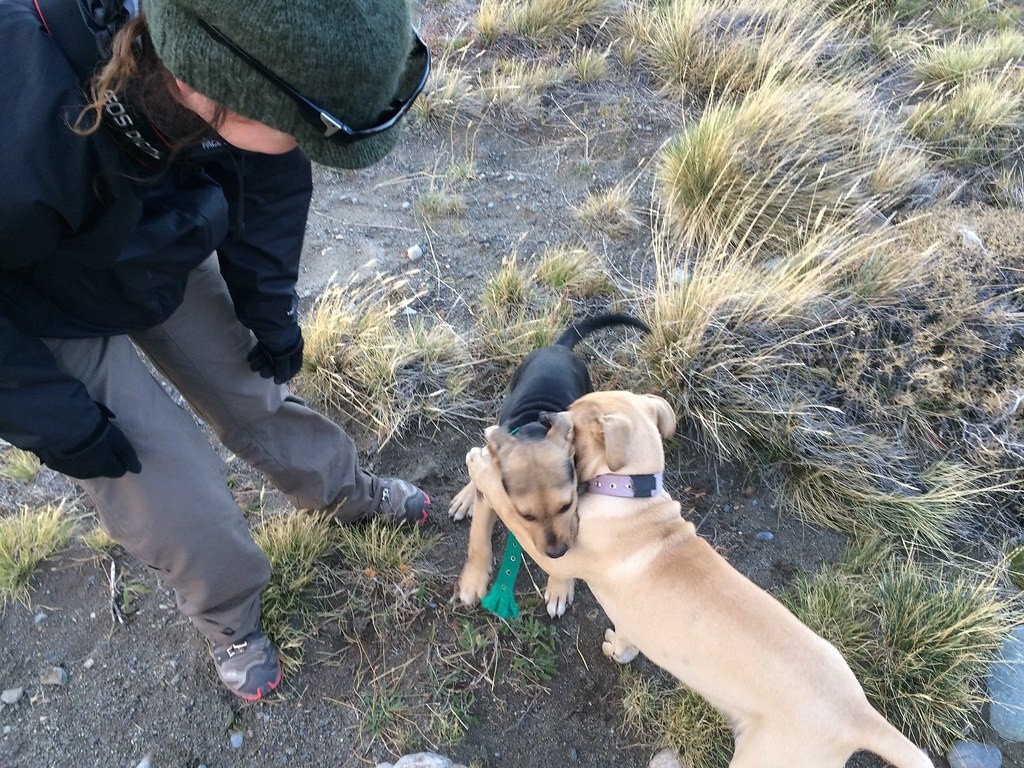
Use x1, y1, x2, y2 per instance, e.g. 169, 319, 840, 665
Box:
206, 627, 283, 700
374, 476, 432, 530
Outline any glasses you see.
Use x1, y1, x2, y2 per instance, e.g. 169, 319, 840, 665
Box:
173, 0, 433, 144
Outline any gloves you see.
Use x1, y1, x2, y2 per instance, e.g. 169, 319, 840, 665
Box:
244, 333, 306, 385
34, 400, 143, 480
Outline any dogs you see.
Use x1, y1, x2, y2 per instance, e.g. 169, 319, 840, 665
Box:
447, 314, 937, 768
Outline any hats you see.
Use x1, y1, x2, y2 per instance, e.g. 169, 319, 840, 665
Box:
140, 0, 412, 170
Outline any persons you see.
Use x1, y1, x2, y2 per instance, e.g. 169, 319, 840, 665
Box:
0, 0, 431, 700
